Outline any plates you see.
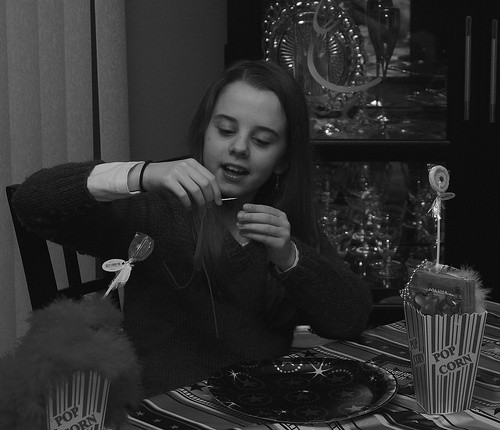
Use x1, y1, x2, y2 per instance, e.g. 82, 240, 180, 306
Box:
207, 357, 399, 423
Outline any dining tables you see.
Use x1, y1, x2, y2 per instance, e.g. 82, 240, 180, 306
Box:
103, 299, 500, 430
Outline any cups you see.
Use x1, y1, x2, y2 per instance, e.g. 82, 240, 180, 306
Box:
328, 220, 354, 256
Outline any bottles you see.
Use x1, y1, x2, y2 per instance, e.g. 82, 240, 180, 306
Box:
342, 163, 379, 201
319, 180, 336, 217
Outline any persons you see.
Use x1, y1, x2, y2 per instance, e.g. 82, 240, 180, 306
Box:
12, 59, 374, 399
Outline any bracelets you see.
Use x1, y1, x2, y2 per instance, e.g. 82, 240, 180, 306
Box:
139, 160, 153, 193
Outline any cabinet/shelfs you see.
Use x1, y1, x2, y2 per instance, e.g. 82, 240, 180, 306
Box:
224, 0, 500, 328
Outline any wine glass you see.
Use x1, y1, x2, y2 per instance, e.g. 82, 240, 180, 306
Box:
366, 0, 401, 112
414, 164, 442, 257
374, 206, 406, 280
356, 193, 406, 282
